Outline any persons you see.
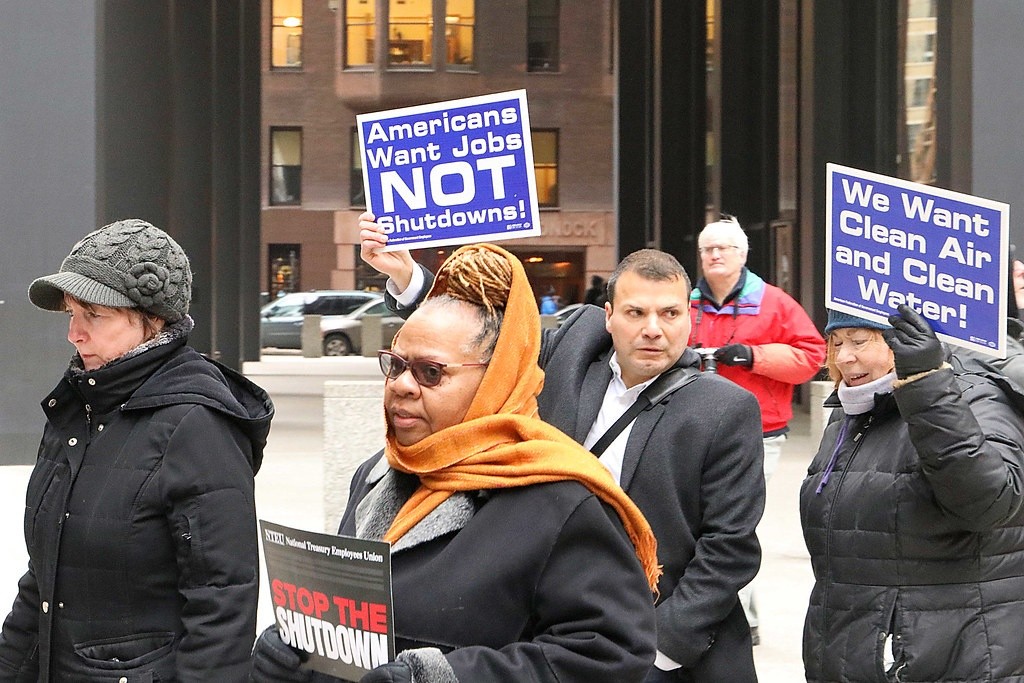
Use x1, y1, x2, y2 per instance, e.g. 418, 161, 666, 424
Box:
537, 287, 558, 317
0, 216, 274, 680
980, 241, 1024, 414
355, 206, 770, 681
578, 272, 604, 310
797, 238, 1024, 683
682, 213, 827, 646
247, 241, 660, 683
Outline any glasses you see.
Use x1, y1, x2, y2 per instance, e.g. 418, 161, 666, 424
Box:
701, 244, 740, 253
377, 348, 488, 389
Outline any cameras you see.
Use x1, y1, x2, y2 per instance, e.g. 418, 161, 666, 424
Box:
692, 347, 720, 373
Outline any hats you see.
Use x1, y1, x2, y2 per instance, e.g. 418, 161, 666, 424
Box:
825, 309, 891, 331
28, 219, 191, 321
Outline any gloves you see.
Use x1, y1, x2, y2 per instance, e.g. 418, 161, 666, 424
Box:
882, 303, 943, 380
249, 623, 308, 682
713, 344, 752, 369
357, 661, 410, 682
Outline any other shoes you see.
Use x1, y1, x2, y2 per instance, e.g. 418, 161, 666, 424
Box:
750, 626, 761, 646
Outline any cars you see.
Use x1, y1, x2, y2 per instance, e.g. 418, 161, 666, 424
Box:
260, 288, 408, 356
